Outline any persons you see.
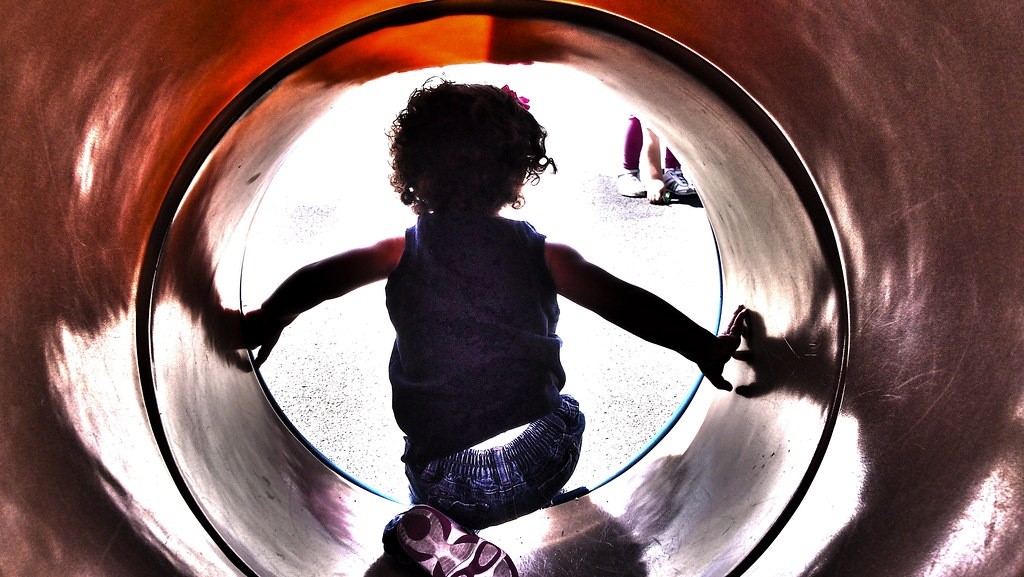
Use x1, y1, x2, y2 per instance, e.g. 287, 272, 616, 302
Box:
219, 76, 749, 577
617, 112, 697, 202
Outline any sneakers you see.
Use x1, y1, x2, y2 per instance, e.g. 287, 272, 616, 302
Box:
617, 168, 647, 197
662, 168, 697, 194
381, 504, 519, 577
